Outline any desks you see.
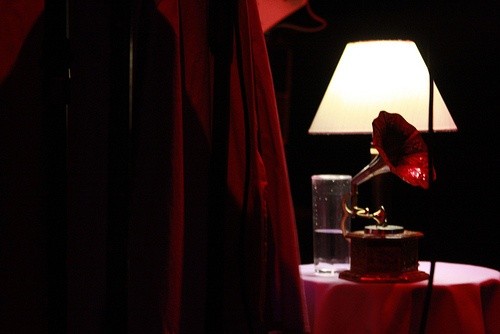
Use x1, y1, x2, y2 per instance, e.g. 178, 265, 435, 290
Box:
298, 260, 499, 334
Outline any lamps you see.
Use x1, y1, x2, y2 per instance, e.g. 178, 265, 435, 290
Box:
307, 39, 459, 225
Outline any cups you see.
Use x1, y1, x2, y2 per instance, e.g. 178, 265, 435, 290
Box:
310, 174, 353, 273
